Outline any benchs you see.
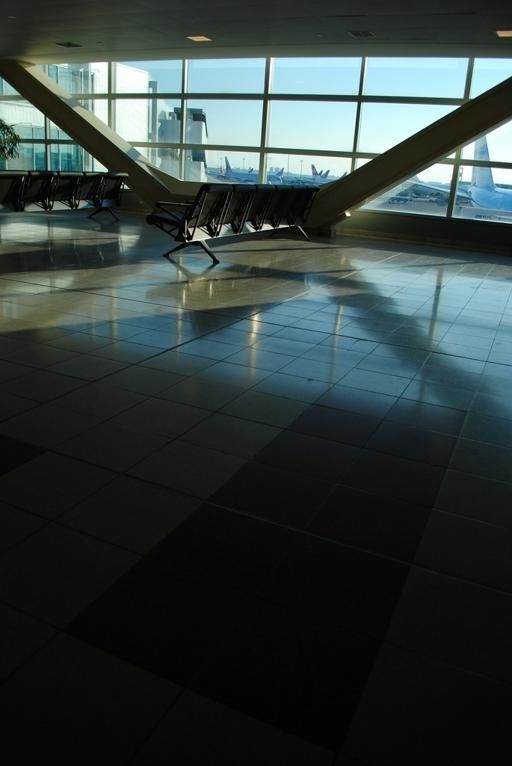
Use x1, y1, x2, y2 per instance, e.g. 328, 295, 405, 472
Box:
144, 181, 320, 264
0, 169, 129, 222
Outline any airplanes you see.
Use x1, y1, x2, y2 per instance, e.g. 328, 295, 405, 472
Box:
205, 135, 512, 222
